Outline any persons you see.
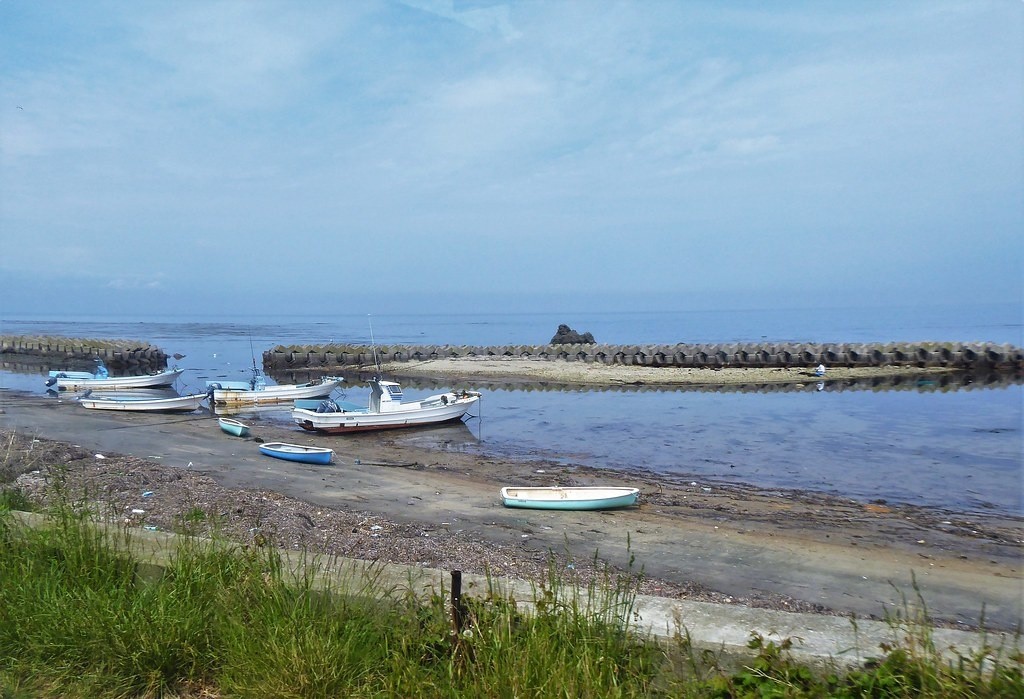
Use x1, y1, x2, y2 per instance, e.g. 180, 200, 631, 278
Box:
816, 381, 824, 391
815, 361, 825, 376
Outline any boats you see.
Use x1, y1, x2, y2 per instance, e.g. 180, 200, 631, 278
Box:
258, 442, 333, 465
79, 391, 208, 413
218, 417, 250, 437
57, 362, 185, 391
499, 487, 640, 511
213, 326, 344, 404
291, 312, 484, 436
